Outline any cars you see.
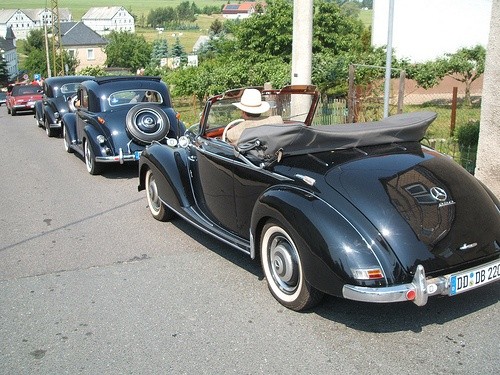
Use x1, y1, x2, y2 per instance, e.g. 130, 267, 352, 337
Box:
4, 84, 44, 117
60, 74, 187, 176
32, 75, 96, 137
0, 92, 7, 107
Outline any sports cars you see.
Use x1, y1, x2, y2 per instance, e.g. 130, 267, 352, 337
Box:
137, 84, 500, 313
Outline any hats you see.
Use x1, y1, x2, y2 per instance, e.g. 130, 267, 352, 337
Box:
232, 89, 270, 114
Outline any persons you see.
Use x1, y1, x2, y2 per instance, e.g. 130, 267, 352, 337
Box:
226, 89, 283, 148
2, 87, 8, 92
7, 84, 14, 92
129, 91, 143, 103
31, 77, 44, 90
75, 90, 88, 108
